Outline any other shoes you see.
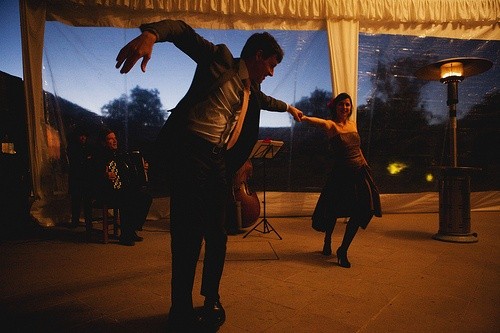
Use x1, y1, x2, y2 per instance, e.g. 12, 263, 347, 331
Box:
132, 231, 144, 242
86, 222, 92, 229
73, 216, 79, 225
119, 234, 135, 246
168, 305, 213, 333
202, 296, 226, 326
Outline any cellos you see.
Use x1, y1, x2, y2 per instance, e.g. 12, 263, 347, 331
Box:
225, 156, 261, 237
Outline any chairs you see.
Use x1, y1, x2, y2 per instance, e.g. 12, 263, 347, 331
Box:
83, 191, 118, 243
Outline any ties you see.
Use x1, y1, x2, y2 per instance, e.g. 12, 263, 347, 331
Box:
225, 79, 250, 151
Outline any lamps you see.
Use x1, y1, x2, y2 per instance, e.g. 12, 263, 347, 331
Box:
414, 56, 496, 244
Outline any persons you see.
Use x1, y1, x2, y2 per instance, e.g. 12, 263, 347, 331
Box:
299, 93, 382, 269
116, 20, 304, 329
63, 125, 151, 246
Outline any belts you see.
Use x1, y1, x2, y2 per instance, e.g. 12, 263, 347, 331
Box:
182, 131, 227, 157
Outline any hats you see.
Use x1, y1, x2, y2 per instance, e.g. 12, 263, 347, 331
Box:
96, 128, 116, 148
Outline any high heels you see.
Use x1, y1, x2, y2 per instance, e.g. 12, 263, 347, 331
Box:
335, 247, 351, 268
321, 238, 331, 256
